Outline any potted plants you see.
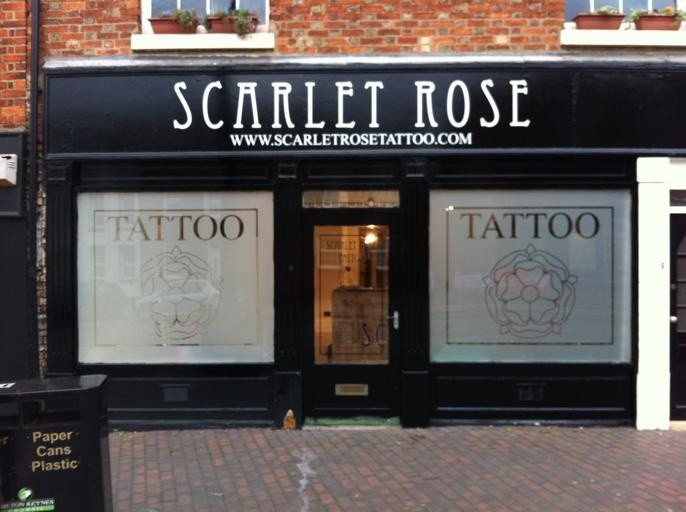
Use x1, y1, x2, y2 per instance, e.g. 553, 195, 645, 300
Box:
208, 10, 258, 34
148, 11, 198, 33
573, 5, 626, 29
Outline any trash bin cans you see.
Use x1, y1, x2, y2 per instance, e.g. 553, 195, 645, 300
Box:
2, 374, 113, 511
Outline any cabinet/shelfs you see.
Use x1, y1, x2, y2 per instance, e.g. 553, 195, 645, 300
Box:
331, 286, 389, 364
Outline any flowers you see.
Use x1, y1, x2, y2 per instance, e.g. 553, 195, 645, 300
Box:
630, 6, 686, 26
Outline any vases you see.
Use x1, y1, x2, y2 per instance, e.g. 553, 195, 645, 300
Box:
635, 15, 681, 30
